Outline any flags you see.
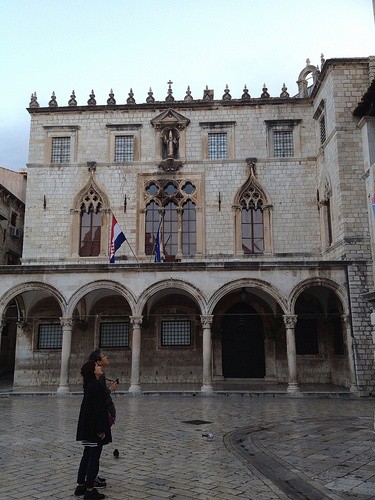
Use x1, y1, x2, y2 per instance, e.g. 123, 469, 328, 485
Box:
110, 214, 126, 263
153, 218, 162, 262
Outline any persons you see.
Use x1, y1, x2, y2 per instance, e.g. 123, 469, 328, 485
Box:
89, 347, 116, 488
74, 362, 118, 500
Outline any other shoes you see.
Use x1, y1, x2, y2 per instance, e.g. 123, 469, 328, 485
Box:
84, 489, 105, 500
74, 485, 85, 495
94, 480, 106, 489
98, 477, 106, 482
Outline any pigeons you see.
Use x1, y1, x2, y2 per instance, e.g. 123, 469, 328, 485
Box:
113, 449, 120, 458
202, 433, 214, 439
